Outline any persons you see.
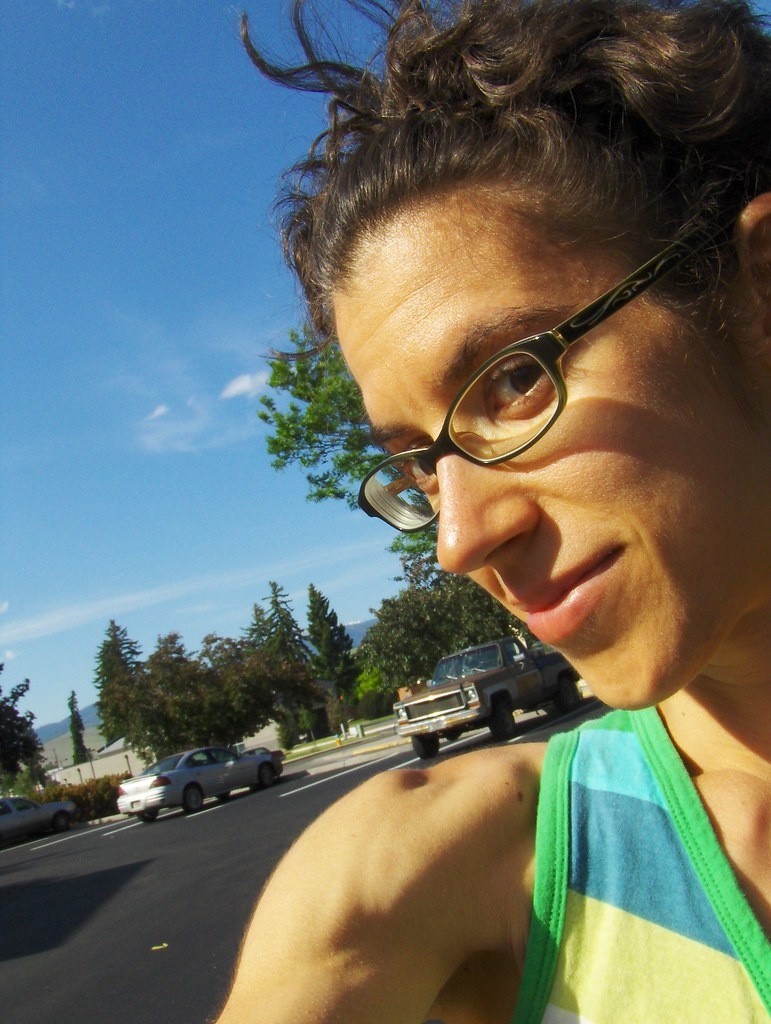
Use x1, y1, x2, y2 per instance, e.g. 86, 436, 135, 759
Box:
216, 0, 770, 1023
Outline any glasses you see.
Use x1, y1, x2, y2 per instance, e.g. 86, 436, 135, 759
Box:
357, 189, 760, 535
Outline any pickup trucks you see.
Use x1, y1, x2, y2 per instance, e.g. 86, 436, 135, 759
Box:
391, 634, 583, 761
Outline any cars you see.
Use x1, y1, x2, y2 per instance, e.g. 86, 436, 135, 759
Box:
115, 745, 284, 822
0, 797, 78, 850
